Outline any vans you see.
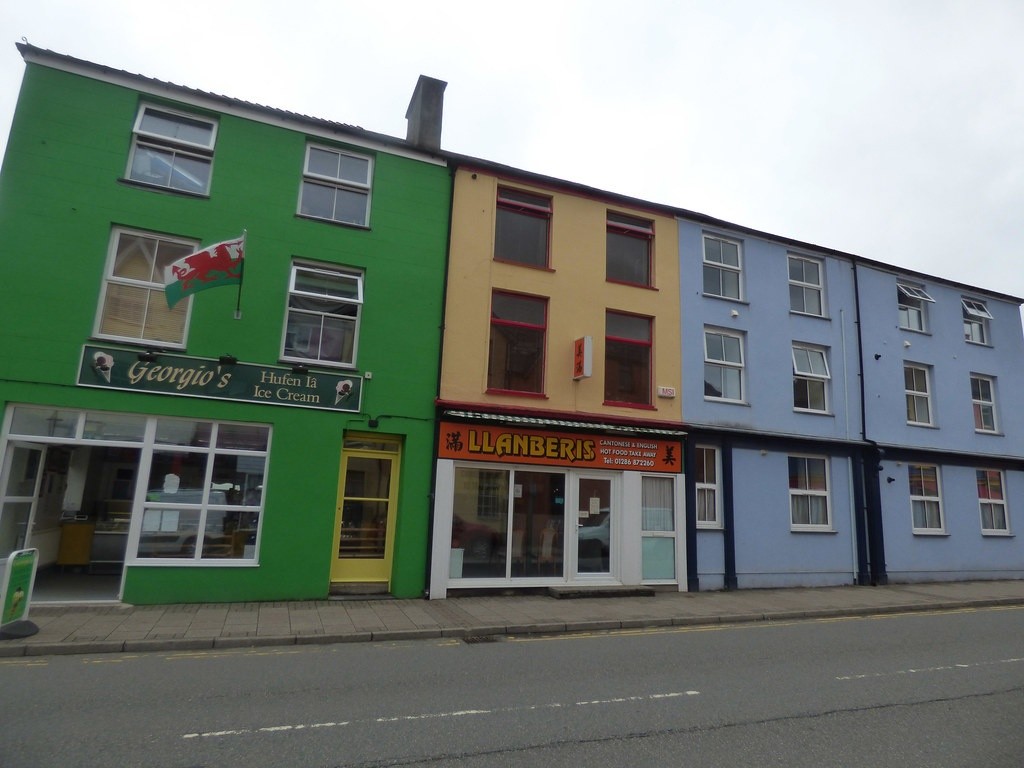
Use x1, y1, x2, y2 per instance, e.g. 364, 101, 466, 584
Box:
141, 489, 230, 558
573, 505, 673, 555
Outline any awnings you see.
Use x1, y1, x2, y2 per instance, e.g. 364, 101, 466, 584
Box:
442, 410, 688, 440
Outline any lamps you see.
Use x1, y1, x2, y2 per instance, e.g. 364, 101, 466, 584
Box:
220, 353, 238, 366
138, 345, 166, 362
290, 365, 310, 375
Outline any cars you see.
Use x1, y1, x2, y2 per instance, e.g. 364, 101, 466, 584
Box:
348, 504, 503, 561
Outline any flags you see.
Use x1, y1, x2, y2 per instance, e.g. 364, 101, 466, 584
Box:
163, 235, 244, 311
